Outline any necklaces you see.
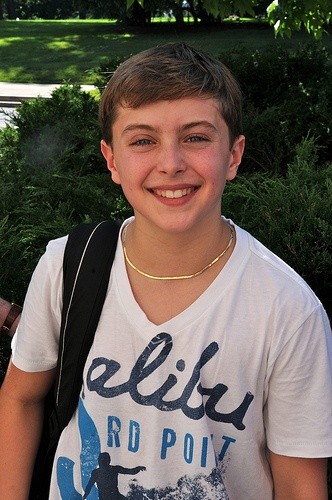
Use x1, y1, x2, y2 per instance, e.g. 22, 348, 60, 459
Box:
123, 216, 233, 280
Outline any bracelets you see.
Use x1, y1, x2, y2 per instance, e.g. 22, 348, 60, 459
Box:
0, 303, 22, 335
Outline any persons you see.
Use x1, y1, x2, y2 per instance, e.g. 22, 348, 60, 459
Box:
0, 43, 332, 500
0, 298, 23, 337
